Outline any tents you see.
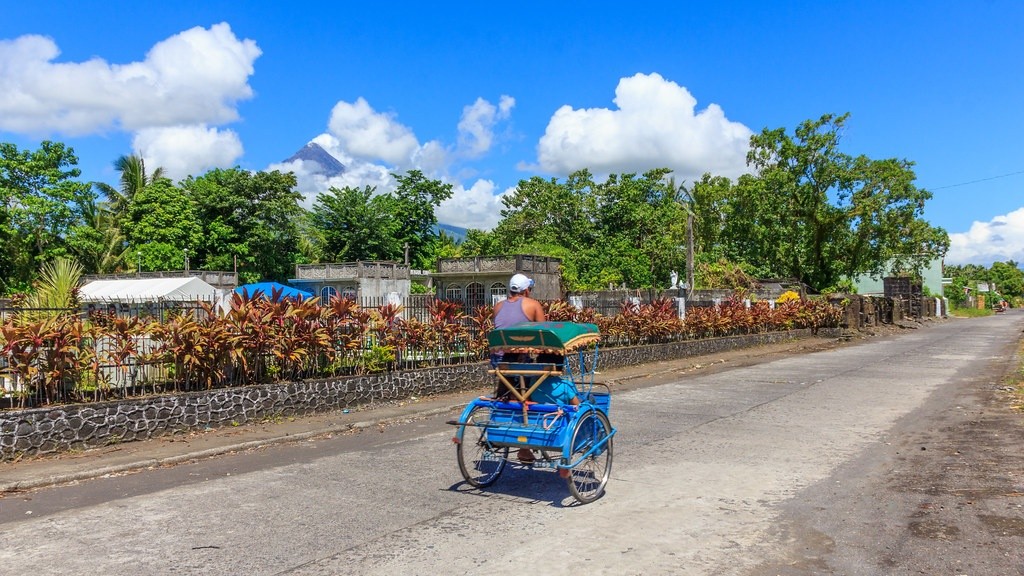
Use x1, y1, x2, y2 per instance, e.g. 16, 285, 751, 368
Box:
76, 277, 224, 327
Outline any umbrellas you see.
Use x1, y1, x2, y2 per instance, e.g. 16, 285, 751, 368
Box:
232, 281, 314, 302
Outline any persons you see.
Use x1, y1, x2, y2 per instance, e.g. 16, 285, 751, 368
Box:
529, 349, 581, 406
493, 275, 545, 460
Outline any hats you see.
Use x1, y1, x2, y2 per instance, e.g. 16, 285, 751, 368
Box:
509, 273, 537, 293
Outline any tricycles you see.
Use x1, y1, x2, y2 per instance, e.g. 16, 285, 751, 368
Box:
995, 307, 1006, 315
443, 322, 616, 504
